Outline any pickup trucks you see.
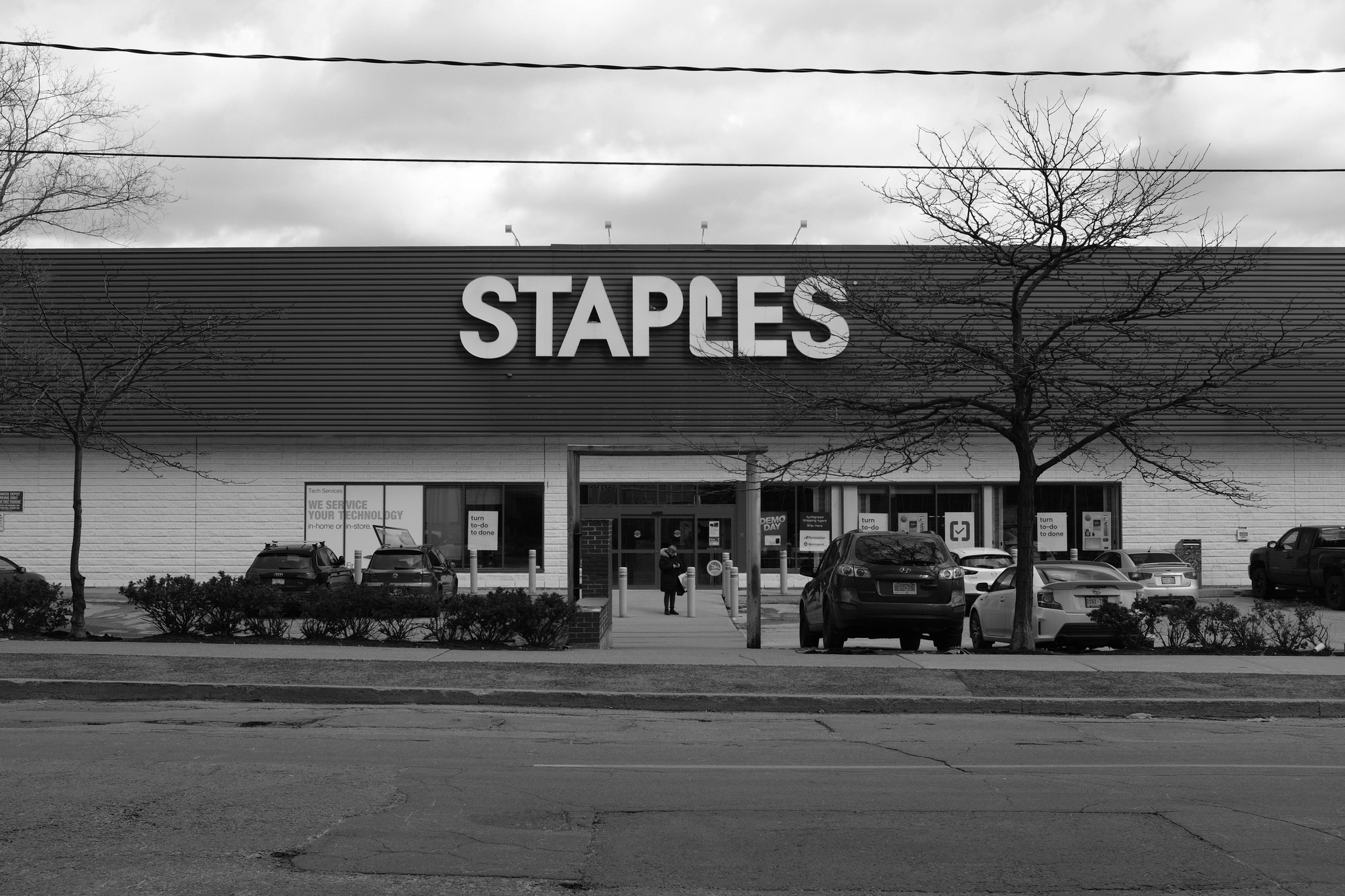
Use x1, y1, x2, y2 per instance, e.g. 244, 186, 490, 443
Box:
1247, 525, 1345, 611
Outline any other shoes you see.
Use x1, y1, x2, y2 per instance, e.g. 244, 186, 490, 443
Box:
665, 609, 670, 615
670, 608, 679, 615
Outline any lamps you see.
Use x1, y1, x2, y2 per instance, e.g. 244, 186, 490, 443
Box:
605, 221, 612, 244
701, 221, 709, 244
792, 219, 807, 244
505, 225, 520, 246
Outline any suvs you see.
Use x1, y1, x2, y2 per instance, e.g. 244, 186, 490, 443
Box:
798, 528, 966, 652
361, 543, 458, 602
245, 540, 355, 618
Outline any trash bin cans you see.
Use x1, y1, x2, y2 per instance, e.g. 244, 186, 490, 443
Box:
1175, 539, 1202, 589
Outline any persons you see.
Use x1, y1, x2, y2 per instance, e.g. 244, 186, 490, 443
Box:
659, 545, 680, 615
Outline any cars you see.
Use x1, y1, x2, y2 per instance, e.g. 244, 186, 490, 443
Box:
948, 548, 1018, 615
969, 560, 1156, 651
1094, 548, 1198, 612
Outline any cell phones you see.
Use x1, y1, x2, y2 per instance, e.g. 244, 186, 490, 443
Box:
676, 564, 679, 566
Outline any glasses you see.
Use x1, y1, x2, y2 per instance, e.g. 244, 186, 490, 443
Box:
670, 551, 677, 554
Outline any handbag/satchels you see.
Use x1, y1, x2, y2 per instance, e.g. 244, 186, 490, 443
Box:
677, 572, 687, 596
